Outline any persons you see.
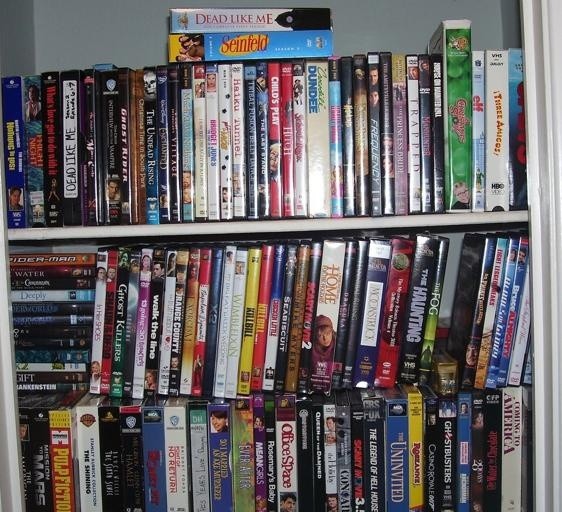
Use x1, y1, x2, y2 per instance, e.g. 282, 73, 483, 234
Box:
451, 181, 470, 209
175, 34, 205, 63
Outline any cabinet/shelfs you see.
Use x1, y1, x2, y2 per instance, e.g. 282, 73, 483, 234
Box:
0, 0, 562, 512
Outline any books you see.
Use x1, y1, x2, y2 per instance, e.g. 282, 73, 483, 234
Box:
89, 230, 534, 399
428, 19, 471, 219
168, 7, 333, 34
508, 48, 529, 212
431, 53, 445, 214
7, 249, 98, 391
472, 50, 487, 211
485, 50, 510, 211
17, 392, 536, 511
2, 54, 429, 227
167, 30, 333, 64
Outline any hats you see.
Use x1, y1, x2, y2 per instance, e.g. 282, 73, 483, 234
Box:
314, 315, 332, 328
